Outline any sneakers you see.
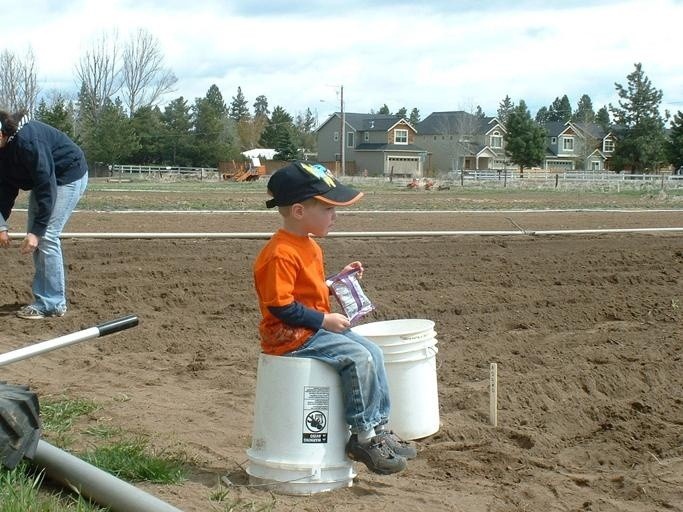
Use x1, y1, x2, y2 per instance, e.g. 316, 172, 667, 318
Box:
15, 304, 64, 319
346, 434, 408, 475
376, 430, 418, 460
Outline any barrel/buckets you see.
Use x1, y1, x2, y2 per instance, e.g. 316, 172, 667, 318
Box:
245, 352, 357, 494
351, 319, 440, 438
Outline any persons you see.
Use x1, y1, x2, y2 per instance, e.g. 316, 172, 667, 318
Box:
252, 162, 417, 476
0, 109, 88, 318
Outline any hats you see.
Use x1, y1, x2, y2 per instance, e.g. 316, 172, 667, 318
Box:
265, 160, 364, 209
